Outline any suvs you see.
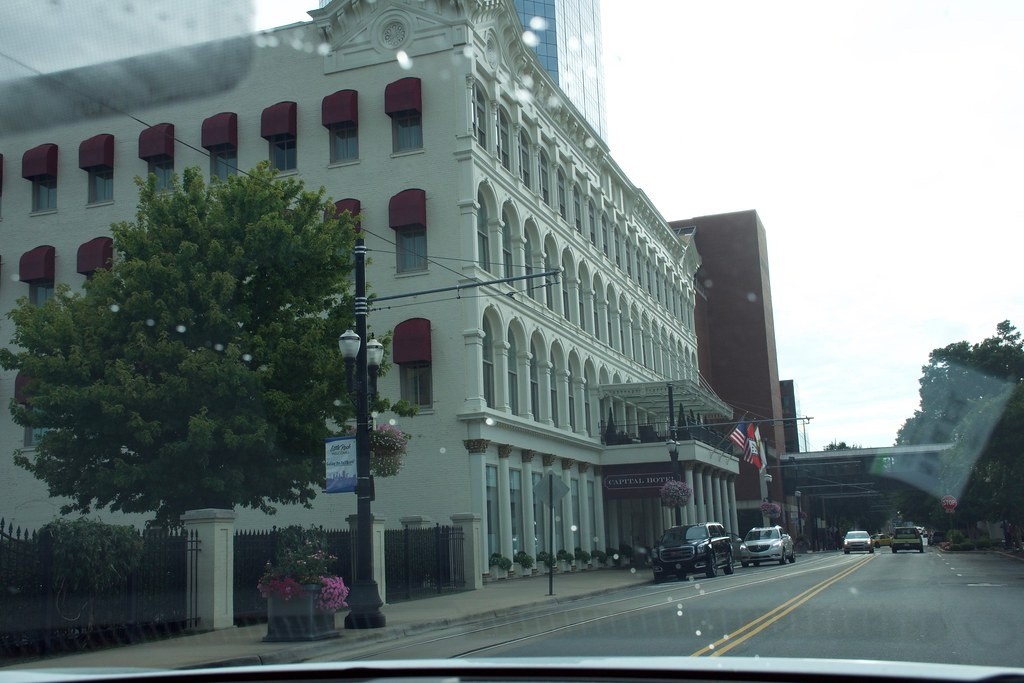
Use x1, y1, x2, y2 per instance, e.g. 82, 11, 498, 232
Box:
650, 522, 735, 583
890, 527, 924, 553
739, 525, 797, 568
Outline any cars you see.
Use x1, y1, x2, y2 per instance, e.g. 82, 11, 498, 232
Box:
871, 534, 893, 548
841, 530, 875, 554
915, 527, 928, 539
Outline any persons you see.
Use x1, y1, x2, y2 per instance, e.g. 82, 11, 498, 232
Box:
729, 416, 748, 452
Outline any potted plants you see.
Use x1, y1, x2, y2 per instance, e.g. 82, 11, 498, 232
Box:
489, 552, 512, 581
557, 549, 573, 573
513, 551, 534, 577
606, 544, 633, 569
591, 550, 608, 570
574, 547, 591, 571
536, 550, 556, 575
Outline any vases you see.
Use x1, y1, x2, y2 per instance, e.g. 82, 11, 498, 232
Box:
260, 584, 340, 642
795, 543, 808, 554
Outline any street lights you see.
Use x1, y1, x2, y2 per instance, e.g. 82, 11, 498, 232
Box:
338, 323, 388, 630
764, 473, 774, 503
665, 439, 683, 523
795, 489, 803, 534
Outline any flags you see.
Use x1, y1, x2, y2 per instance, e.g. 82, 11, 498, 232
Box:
744, 423, 762, 468
754, 425, 767, 473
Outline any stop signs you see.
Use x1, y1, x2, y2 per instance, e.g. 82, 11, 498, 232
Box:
941, 495, 957, 510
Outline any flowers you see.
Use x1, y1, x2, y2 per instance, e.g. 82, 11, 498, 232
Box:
660, 479, 693, 507
794, 534, 811, 549
761, 502, 781, 519
800, 511, 808, 520
256, 525, 350, 610
345, 426, 411, 478
